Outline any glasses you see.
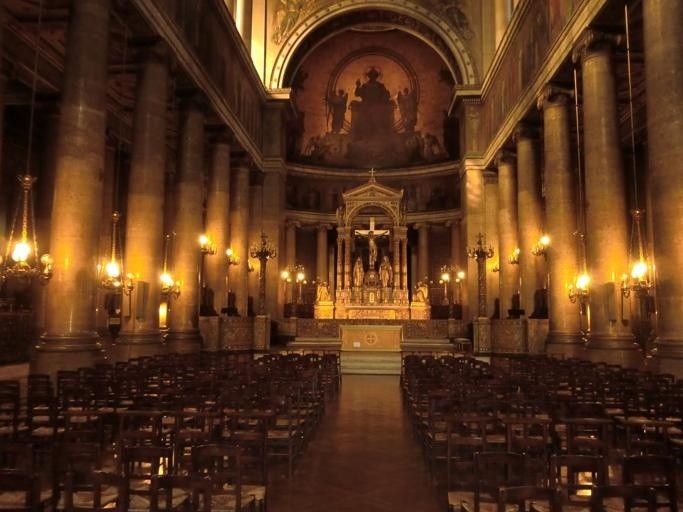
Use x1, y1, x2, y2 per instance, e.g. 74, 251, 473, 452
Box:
190, 230, 310, 326
91, 22, 140, 297
434, 231, 553, 318
557, 60, 602, 351
0, 1, 55, 297
600, 0, 673, 368
154, 50, 180, 309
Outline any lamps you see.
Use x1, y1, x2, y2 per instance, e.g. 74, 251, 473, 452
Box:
434, 231, 553, 318
0, 1, 55, 297
600, 0, 673, 368
557, 60, 602, 351
154, 50, 180, 309
91, 22, 140, 297
190, 230, 310, 326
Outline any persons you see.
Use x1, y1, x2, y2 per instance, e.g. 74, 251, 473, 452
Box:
348, 67, 398, 128
414, 282, 428, 302
356, 230, 388, 269
396, 88, 418, 129
316, 282, 332, 301
379, 256, 393, 287
353, 257, 364, 285
328, 89, 349, 134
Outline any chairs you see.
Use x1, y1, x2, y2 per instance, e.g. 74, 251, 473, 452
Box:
396, 346, 682, 512
0, 345, 343, 510
